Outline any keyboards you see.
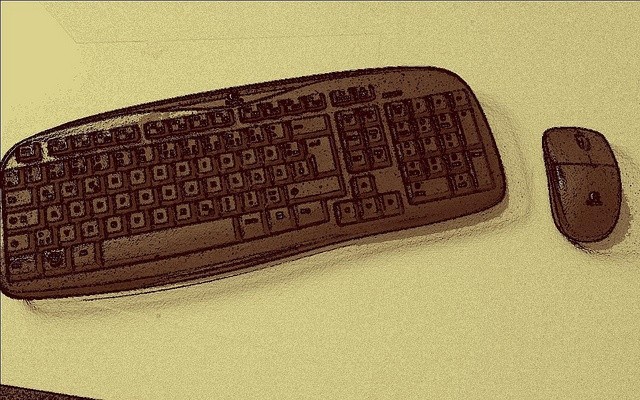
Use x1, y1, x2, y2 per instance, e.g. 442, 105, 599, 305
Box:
0, 66, 506, 299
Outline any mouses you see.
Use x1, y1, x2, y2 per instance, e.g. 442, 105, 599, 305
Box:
542, 127, 623, 242
